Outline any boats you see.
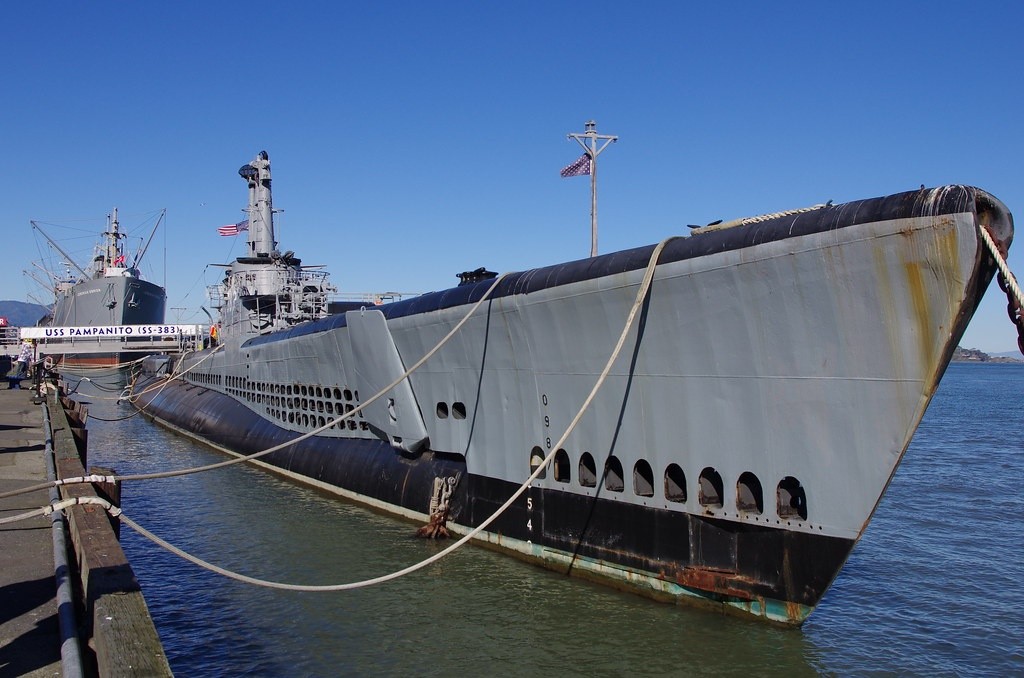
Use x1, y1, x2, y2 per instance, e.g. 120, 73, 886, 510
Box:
129, 118, 1016, 633
20, 206, 171, 372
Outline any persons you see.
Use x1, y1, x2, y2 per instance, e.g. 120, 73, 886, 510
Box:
210, 322, 219, 347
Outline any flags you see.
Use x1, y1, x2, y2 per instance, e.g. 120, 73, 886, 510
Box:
560, 153, 592, 177
113, 255, 125, 265
218, 219, 250, 236
